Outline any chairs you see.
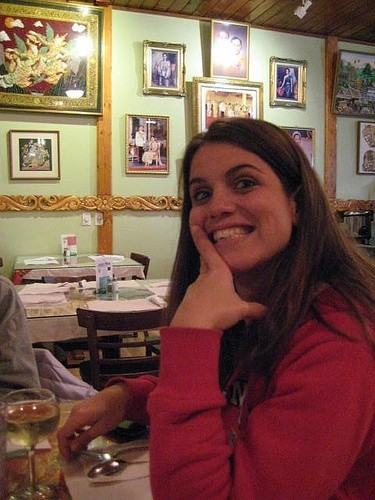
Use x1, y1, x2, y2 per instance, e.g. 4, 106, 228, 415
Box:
121, 252, 151, 280
76, 308, 168, 393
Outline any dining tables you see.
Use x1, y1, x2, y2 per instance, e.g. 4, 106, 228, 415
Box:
0, 398, 155, 500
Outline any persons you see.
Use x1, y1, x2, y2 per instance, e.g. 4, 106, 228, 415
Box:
0, 275, 42, 407
56, 119, 375, 500
136, 31, 313, 167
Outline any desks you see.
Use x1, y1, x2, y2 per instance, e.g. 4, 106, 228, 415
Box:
13, 277, 171, 384
13, 252, 145, 285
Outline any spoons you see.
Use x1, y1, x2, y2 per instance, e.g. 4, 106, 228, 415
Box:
87, 458, 149, 477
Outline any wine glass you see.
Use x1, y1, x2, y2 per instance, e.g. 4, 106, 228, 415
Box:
3, 387, 61, 500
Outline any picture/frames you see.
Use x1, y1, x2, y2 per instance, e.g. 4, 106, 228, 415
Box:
269, 56, 307, 108
210, 19, 251, 81
9, 129, 60, 180
356, 121, 375, 175
280, 126, 315, 171
191, 77, 263, 139
125, 113, 170, 175
331, 48, 375, 118
142, 40, 187, 97
0, 0, 107, 116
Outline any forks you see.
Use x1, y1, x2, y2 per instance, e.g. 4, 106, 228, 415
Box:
78, 441, 148, 464
